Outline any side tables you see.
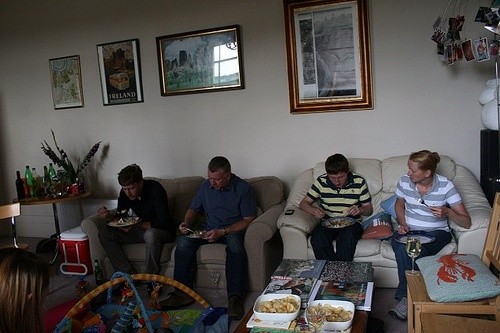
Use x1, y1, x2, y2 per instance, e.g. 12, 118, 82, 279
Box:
480, 129, 500, 209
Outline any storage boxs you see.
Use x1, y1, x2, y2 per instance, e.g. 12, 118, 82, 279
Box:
58, 225, 93, 275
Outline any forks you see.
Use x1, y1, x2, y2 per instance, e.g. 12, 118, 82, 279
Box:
416, 197, 434, 211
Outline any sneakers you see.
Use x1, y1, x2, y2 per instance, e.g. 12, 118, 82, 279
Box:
387, 296, 408, 320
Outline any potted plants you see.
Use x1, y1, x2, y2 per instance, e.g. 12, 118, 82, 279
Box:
40, 128, 102, 194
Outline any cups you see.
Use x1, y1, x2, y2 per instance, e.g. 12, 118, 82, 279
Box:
306, 307, 325, 333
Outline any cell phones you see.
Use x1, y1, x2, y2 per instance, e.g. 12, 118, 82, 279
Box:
285, 210, 295, 215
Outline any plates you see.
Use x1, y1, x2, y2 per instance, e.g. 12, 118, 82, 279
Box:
324, 216, 356, 228
184, 229, 211, 240
106, 217, 142, 227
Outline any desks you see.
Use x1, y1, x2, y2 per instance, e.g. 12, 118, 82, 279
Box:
13, 186, 92, 239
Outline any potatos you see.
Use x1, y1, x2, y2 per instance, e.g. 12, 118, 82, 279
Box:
308, 303, 352, 322
260, 294, 299, 313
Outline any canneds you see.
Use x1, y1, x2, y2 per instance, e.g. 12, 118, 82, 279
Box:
72, 184, 79, 196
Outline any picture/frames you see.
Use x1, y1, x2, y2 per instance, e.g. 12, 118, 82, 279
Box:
283, 0, 375, 115
96, 40, 145, 106
154, 24, 246, 97
48, 54, 85, 110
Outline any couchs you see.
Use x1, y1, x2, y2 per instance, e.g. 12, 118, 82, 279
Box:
276, 154, 494, 289
79, 175, 288, 292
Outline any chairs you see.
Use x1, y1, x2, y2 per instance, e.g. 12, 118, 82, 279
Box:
0, 202, 29, 251
404, 192, 500, 333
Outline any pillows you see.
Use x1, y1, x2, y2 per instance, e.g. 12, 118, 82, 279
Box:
415, 253, 500, 303
360, 211, 394, 240
379, 194, 401, 226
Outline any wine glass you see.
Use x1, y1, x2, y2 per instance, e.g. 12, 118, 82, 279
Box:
405, 235, 422, 277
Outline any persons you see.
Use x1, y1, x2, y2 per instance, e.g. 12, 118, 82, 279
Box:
96, 163, 177, 276
299, 153, 374, 263
160, 156, 257, 321
279, 277, 306, 298
0, 245, 52, 333
388, 149, 472, 321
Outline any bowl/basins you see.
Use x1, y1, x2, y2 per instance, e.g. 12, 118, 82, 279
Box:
304, 300, 355, 331
253, 294, 301, 321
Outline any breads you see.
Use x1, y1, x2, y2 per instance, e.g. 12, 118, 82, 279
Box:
343, 209, 348, 217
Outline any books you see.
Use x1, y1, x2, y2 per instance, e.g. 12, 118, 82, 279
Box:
259, 270, 317, 308
307, 279, 374, 312
245, 312, 291, 329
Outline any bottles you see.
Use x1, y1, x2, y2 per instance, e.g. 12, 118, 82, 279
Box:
15, 170, 25, 199
48, 163, 57, 180
43, 166, 50, 184
94, 259, 103, 285
30, 167, 39, 185
25, 166, 34, 198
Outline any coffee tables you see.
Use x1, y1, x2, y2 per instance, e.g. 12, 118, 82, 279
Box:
233, 258, 375, 333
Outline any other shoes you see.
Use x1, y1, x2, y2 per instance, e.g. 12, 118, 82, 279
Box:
228, 294, 245, 320
147, 281, 156, 293
159, 290, 198, 308
